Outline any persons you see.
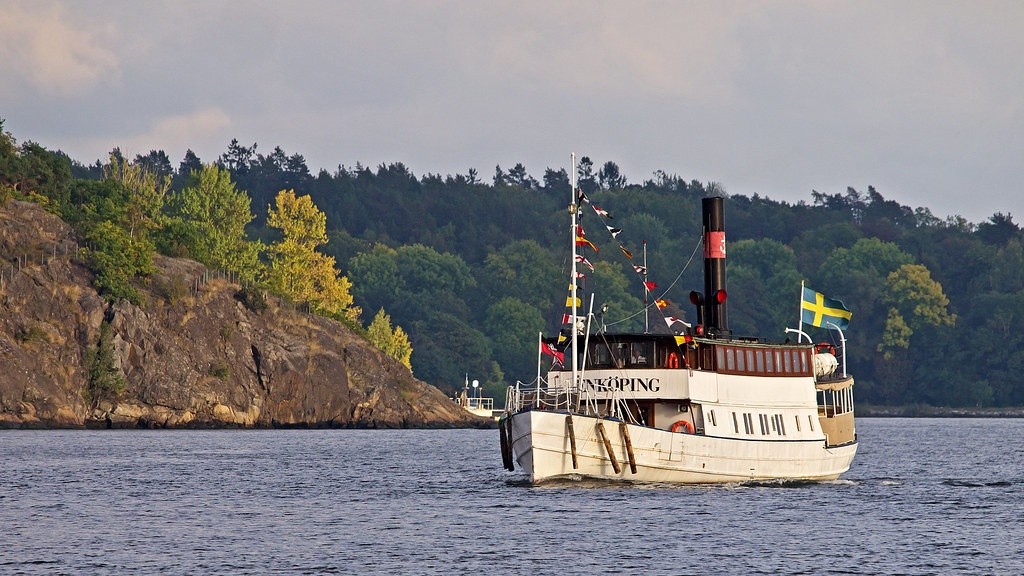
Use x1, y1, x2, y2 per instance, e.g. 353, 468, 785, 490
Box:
631, 351, 647, 365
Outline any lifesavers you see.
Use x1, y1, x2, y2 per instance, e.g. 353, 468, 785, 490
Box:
671, 420, 694, 434
668, 351, 679, 369
814, 342, 836, 356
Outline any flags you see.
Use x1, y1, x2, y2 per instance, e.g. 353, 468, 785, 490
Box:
577, 187, 590, 207
633, 264, 647, 273
565, 296, 582, 307
803, 287, 852, 331
606, 225, 625, 239
664, 316, 692, 329
569, 284, 583, 291
577, 224, 586, 238
575, 236, 600, 253
577, 206, 585, 221
562, 313, 574, 324
540, 335, 567, 363
654, 298, 673, 310
571, 271, 586, 279
558, 329, 573, 343
575, 254, 595, 273
620, 245, 634, 260
643, 281, 659, 292
673, 335, 695, 346
591, 204, 613, 219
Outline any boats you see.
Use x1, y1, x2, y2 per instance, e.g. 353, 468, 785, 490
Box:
493, 152, 858, 487
